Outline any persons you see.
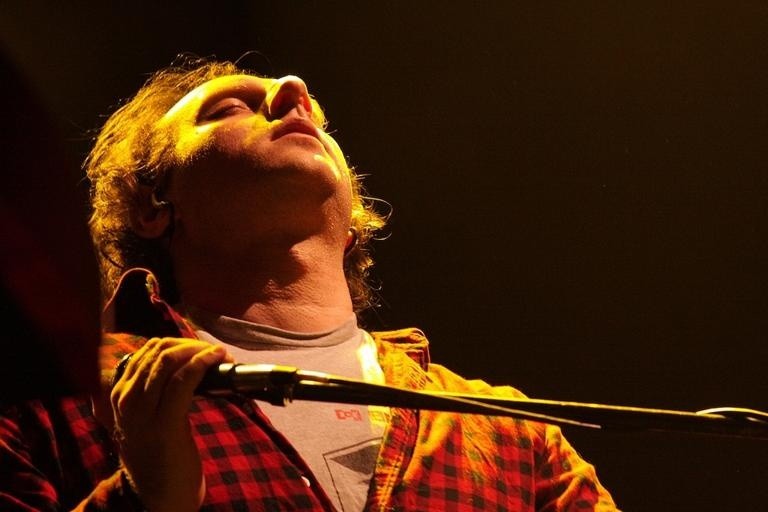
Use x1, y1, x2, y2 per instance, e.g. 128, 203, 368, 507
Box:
2, 57, 625, 512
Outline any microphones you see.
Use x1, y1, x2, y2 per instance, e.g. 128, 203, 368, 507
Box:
112, 352, 298, 389
